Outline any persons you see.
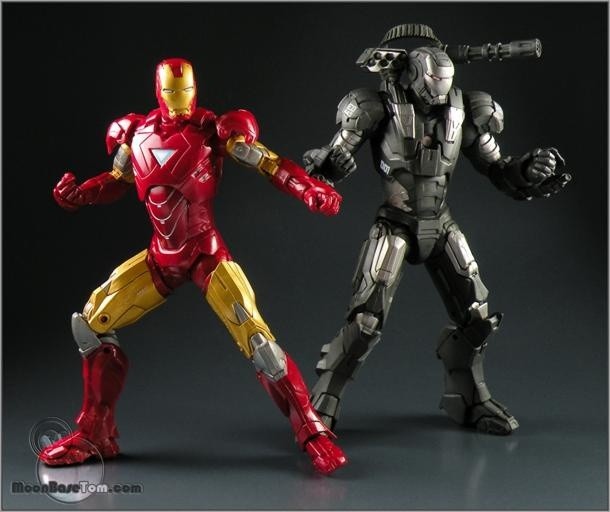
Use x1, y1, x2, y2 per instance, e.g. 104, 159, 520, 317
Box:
38, 58, 352, 473
